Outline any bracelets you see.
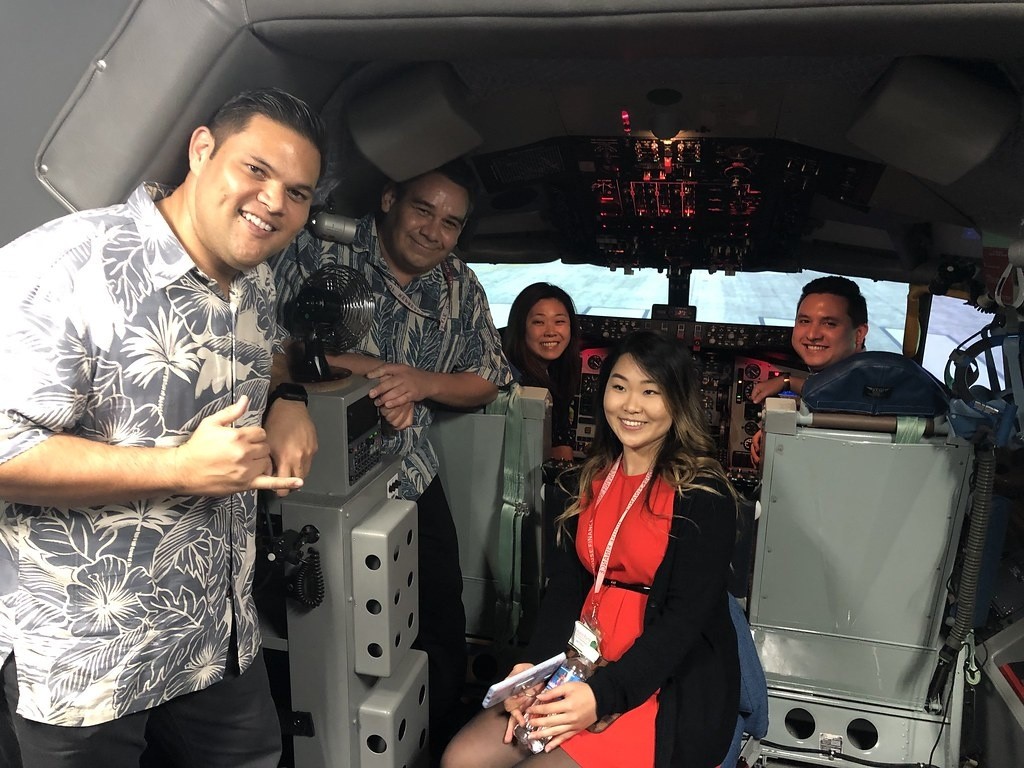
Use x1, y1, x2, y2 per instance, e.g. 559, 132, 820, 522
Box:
782, 373, 790, 392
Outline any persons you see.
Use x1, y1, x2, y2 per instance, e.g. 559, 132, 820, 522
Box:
437, 328, 741, 768
265, 155, 513, 726
0, 85, 327, 768
745, 275, 868, 467
499, 278, 587, 560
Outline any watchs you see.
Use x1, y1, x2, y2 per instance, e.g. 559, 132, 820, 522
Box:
261, 381, 310, 425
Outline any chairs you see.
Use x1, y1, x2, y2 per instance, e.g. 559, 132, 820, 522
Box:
748, 352, 978, 768
418, 386, 555, 671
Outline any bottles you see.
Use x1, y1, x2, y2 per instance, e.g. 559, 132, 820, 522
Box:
512, 645, 600, 754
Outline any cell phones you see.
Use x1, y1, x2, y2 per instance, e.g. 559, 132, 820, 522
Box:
483, 653, 566, 709
